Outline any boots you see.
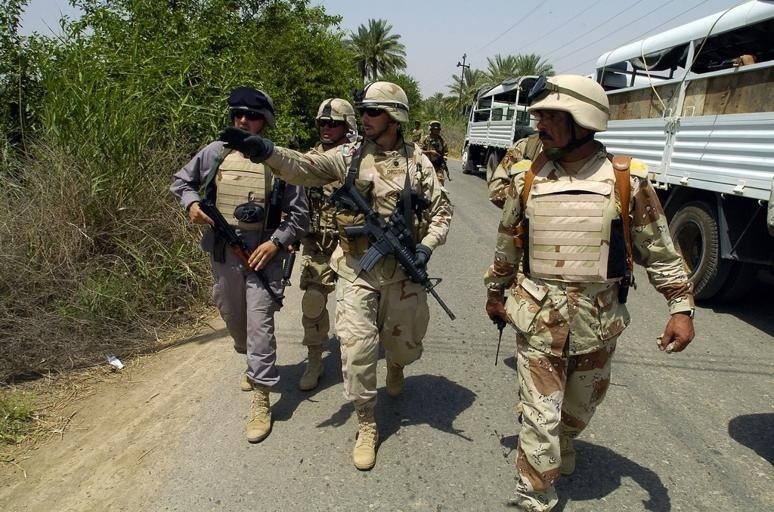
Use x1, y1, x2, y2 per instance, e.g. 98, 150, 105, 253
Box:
247, 383, 272, 442
354, 408, 379, 470
300, 346, 324, 389
386, 365, 404, 396
240, 373, 253, 391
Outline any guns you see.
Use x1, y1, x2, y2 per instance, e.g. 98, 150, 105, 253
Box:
332, 181, 455, 321
200, 199, 282, 303
708, 52, 761, 69
282, 240, 301, 294
432, 141, 451, 181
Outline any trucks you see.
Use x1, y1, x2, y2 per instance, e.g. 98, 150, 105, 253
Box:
459, 74, 543, 184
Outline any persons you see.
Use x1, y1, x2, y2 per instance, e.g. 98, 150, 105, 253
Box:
409, 121, 422, 143
418, 120, 446, 186
482, 72, 696, 510
218, 82, 453, 471
298, 98, 361, 394
168, 86, 307, 447
486, 134, 549, 291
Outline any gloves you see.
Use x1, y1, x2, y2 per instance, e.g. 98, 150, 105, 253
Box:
403, 243, 432, 284
220, 127, 273, 163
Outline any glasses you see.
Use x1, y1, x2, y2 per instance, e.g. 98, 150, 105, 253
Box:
232, 109, 263, 120
358, 107, 386, 117
318, 120, 343, 127
529, 74, 609, 114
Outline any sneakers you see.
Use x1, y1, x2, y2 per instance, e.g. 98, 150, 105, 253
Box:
558, 434, 575, 474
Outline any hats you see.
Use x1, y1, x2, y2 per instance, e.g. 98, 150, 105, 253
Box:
429, 121, 441, 130
527, 75, 609, 131
356, 81, 409, 123
227, 87, 275, 129
315, 97, 357, 130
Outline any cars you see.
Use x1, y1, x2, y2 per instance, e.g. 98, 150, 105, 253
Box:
585, 1, 773, 301
